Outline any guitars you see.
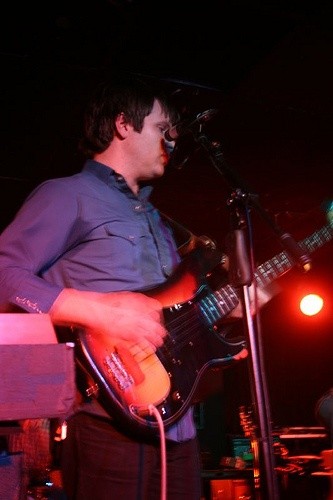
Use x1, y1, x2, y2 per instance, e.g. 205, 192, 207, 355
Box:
74, 194, 332, 431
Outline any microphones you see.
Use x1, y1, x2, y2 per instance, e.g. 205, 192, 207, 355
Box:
164, 108, 217, 142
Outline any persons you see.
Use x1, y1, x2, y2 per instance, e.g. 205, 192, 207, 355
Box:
0, 85, 256, 499
